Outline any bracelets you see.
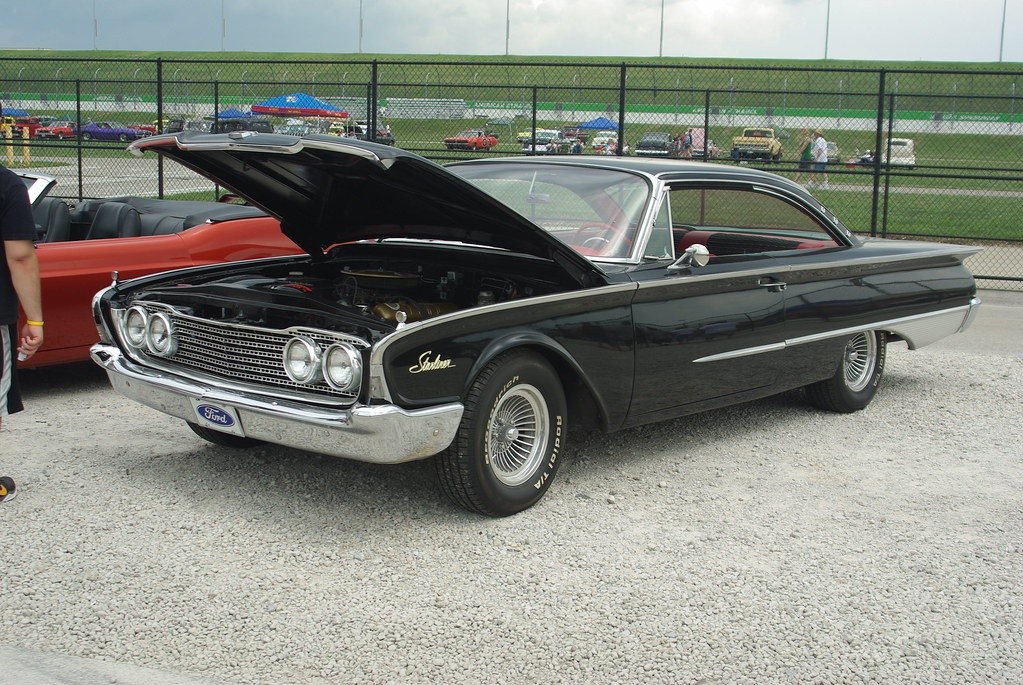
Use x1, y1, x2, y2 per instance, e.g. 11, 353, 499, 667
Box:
26, 319, 45, 325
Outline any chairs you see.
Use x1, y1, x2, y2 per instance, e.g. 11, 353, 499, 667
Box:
84, 201, 141, 240
26, 197, 72, 244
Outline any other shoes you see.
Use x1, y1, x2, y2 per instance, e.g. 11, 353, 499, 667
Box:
0, 476, 17, 503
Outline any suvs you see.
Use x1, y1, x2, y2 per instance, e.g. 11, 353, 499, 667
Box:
199, 118, 274, 134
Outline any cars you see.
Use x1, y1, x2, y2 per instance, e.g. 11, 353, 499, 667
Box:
126, 117, 199, 135
84, 129, 983, 518
0, 107, 57, 140
591, 130, 618, 148
73, 121, 144, 142
635, 131, 675, 158
517, 127, 545, 143
33, 120, 77, 140
274, 118, 397, 148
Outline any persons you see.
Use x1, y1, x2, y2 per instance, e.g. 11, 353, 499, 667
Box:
665, 127, 693, 158
856, 146, 876, 163
545, 138, 586, 154
599, 138, 630, 155
0, 100, 45, 505
805, 127, 831, 190
791, 125, 824, 190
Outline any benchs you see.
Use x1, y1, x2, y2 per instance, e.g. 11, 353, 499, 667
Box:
674, 227, 825, 257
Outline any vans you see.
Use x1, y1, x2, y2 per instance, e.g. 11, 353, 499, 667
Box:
811, 141, 841, 162
527, 129, 564, 146
882, 138, 917, 170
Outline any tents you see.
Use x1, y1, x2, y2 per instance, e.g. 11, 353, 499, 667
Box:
203, 92, 349, 134
486, 115, 518, 142
576, 115, 619, 138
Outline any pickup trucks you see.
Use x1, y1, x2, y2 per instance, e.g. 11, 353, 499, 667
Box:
443, 129, 499, 152
730, 126, 784, 163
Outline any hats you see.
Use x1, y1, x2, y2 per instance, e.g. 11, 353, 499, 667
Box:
814, 128, 823, 136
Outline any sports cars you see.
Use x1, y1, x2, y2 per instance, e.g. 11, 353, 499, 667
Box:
1, 166, 378, 372
